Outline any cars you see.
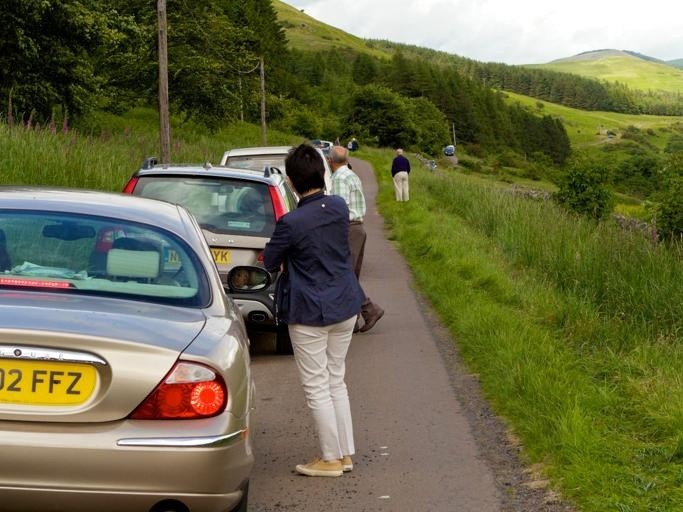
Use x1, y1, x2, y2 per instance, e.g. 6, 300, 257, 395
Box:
0, 184, 256, 512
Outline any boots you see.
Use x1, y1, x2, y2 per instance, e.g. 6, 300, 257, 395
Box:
354, 298, 384, 333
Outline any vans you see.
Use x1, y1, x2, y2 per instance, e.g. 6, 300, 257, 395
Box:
216, 144, 335, 202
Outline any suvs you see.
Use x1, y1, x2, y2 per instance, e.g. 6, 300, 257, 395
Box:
120, 155, 303, 357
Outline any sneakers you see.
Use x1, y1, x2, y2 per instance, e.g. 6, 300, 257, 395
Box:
295, 457, 352, 477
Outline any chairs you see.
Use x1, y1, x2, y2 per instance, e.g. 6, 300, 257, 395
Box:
89, 249, 161, 284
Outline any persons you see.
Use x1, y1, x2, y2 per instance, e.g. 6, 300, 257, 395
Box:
325, 145, 384, 333
333, 136, 359, 152
233, 270, 249, 290
262, 143, 365, 480
391, 149, 410, 201
